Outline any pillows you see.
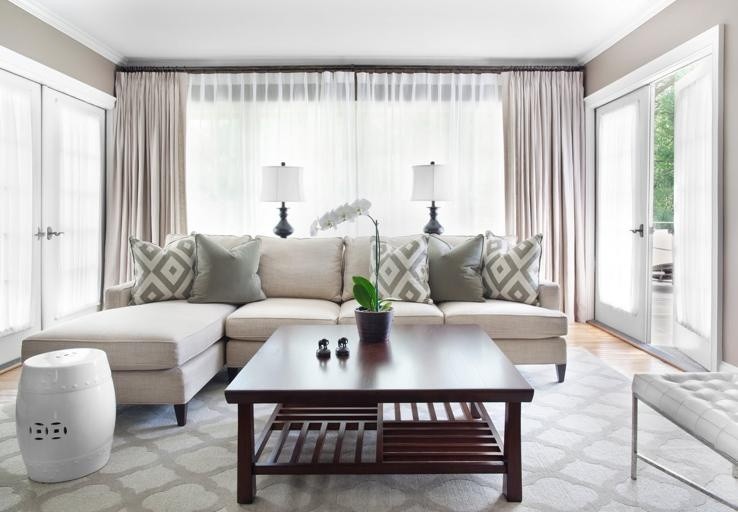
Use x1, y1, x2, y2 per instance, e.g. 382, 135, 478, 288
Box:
370, 240, 433, 305
483, 233, 543, 307
427, 235, 486, 303
187, 234, 267, 303
129, 236, 196, 306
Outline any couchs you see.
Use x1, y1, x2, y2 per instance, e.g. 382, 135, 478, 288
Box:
226, 244, 568, 383
22, 279, 237, 426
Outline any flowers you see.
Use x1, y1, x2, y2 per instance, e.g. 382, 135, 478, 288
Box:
309, 197, 392, 312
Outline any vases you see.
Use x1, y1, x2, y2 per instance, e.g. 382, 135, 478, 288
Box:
355, 306, 394, 342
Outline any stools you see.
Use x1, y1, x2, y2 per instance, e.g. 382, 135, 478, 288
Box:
16, 348, 117, 484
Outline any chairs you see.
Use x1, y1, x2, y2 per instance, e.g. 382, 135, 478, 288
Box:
631, 371, 738, 512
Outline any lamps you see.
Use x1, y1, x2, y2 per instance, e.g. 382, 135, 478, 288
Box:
260, 162, 308, 238
410, 163, 460, 235
